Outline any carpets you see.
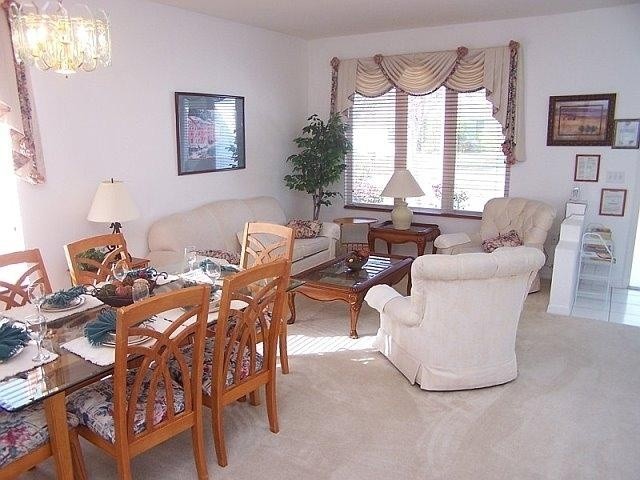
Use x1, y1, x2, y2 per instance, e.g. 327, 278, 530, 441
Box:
21, 274, 640, 479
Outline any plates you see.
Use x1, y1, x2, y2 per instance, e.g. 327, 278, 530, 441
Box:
40, 294, 86, 312
99, 325, 155, 348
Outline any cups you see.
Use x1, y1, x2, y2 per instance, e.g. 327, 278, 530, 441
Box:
133, 282, 150, 303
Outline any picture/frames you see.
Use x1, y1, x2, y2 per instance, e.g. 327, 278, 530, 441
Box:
545, 91, 618, 148
172, 92, 251, 174
574, 153, 601, 182
611, 119, 640, 151
600, 188, 627, 217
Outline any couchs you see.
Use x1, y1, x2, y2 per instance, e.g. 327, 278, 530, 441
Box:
149, 194, 339, 275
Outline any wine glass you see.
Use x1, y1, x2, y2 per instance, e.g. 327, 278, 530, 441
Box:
28, 283, 47, 322
184, 246, 197, 275
205, 262, 221, 292
25, 315, 51, 362
112, 260, 128, 284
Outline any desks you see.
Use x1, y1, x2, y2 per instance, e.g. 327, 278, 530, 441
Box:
333, 217, 378, 252
367, 221, 440, 257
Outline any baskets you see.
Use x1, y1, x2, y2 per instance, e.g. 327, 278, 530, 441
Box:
81, 268, 167, 307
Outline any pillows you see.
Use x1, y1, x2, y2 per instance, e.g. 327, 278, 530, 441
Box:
477, 230, 521, 252
285, 218, 324, 239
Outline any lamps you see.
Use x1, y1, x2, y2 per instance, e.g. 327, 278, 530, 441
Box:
89, 179, 146, 262
380, 171, 424, 230
7, 3, 119, 78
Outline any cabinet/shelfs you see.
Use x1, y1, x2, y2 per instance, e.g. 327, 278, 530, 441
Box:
574, 221, 616, 308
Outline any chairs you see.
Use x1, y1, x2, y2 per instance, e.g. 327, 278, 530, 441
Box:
363, 246, 546, 392
434, 198, 560, 293
